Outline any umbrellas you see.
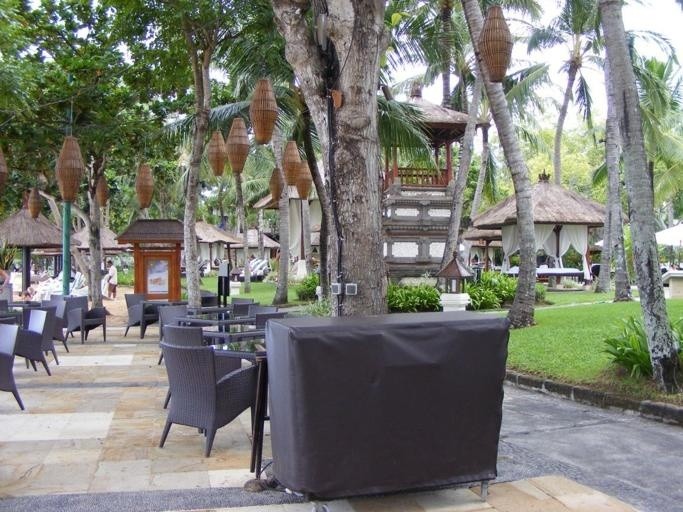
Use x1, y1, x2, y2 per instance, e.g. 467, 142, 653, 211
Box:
657, 222, 683, 268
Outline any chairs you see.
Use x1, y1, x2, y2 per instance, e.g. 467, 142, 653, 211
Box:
0, 293, 109, 409
120, 290, 290, 483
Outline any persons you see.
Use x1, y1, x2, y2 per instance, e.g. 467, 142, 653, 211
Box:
0, 266, 10, 293
106, 258, 118, 299
19, 290, 37, 301
